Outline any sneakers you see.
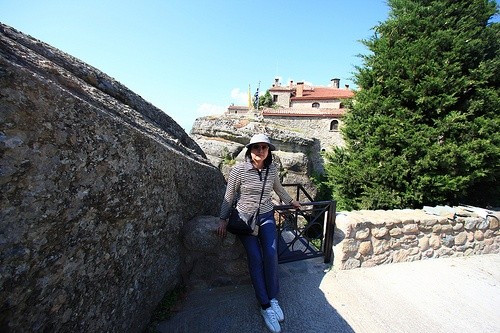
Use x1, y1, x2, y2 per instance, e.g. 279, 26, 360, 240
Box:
269, 298, 285, 322
261, 306, 281, 333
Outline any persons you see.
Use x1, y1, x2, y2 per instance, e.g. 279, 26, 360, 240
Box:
216, 133, 302, 333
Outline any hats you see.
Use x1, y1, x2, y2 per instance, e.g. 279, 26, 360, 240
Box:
245, 134, 275, 151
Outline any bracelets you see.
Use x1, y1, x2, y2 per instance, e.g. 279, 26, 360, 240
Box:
289, 200, 295, 205
220, 217, 226, 221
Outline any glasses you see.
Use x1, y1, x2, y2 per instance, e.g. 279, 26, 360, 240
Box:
251, 144, 270, 151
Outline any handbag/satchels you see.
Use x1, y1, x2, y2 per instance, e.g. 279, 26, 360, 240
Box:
226, 207, 260, 237
